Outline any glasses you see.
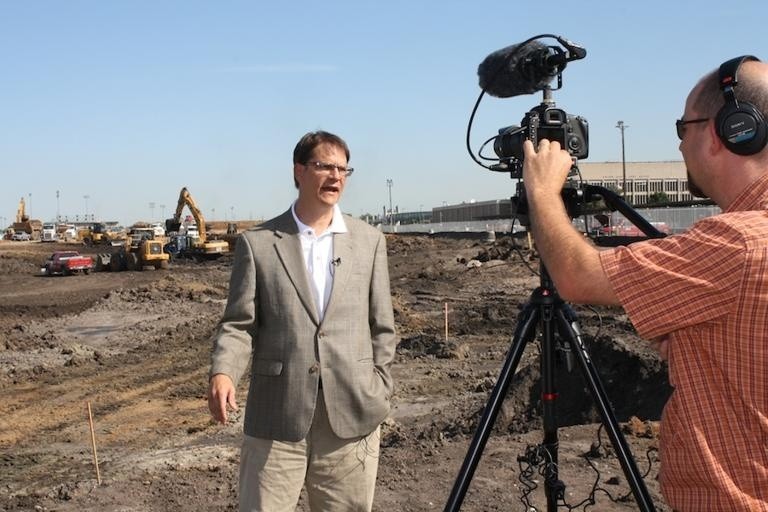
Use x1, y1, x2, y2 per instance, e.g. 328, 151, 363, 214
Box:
677, 118, 708, 140
305, 161, 353, 178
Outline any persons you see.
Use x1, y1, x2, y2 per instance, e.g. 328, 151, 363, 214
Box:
209, 130, 397, 511
522, 58, 768, 511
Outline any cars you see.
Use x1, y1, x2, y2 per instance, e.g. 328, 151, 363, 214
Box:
591, 215, 670, 238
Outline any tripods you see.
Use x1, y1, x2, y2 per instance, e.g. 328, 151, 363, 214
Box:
444, 183, 660, 512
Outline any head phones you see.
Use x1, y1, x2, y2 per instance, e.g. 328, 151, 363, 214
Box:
714, 55, 766, 156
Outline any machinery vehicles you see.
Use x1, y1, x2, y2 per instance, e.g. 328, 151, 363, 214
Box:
4, 188, 230, 278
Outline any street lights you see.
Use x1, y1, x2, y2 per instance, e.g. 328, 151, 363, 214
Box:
613, 119, 630, 201
386, 177, 395, 227
